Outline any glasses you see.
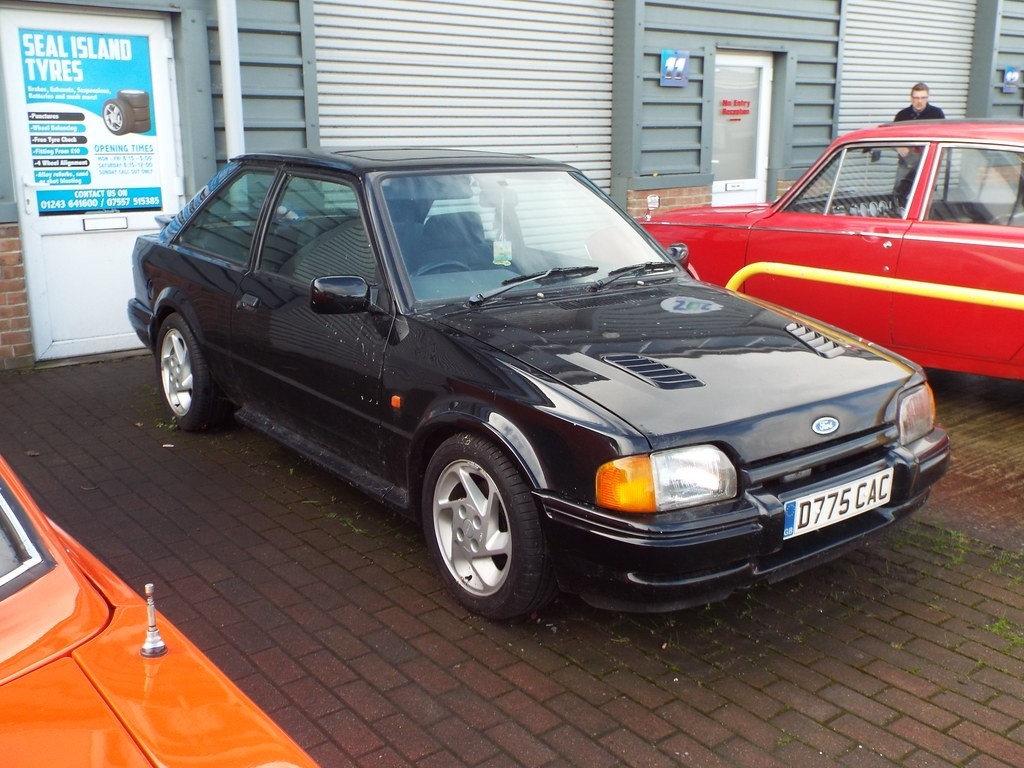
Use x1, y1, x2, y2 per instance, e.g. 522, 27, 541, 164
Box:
912, 95, 929, 100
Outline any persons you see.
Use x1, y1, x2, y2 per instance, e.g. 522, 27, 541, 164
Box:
892, 83, 946, 207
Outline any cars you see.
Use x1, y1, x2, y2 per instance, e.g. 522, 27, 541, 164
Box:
587, 117, 1024, 383
124, 142, 951, 621
0, 451, 323, 768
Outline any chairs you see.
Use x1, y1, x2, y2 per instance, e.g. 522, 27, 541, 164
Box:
419, 210, 496, 281
